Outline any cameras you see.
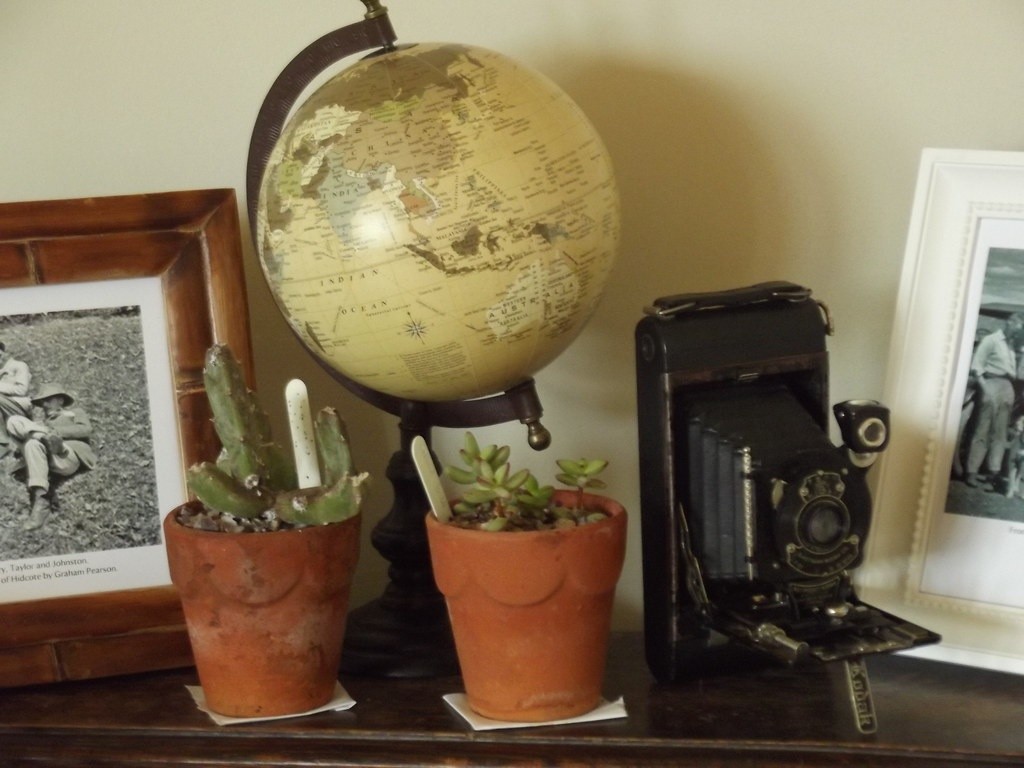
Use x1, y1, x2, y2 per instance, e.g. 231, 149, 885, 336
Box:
632, 282, 942, 683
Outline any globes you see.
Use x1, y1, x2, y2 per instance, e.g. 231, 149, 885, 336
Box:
245, 0, 622, 681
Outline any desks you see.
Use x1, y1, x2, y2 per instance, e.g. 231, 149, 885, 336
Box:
0, 603, 1024, 768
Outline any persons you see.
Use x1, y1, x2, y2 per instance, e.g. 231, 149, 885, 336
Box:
964, 313, 1024, 488
0, 342, 96, 529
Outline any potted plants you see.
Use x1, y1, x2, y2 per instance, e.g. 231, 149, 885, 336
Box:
409, 432, 628, 721
162, 344, 371, 710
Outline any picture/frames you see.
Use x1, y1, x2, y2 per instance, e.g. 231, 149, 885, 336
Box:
0, 187, 271, 693
845, 147, 1024, 676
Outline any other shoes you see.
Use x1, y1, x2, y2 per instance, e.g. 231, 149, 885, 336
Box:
44, 434, 68, 458
24, 501, 51, 530
964, 473, 977, 487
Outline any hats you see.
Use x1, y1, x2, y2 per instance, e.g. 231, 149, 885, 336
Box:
30, 382, 74, 408
